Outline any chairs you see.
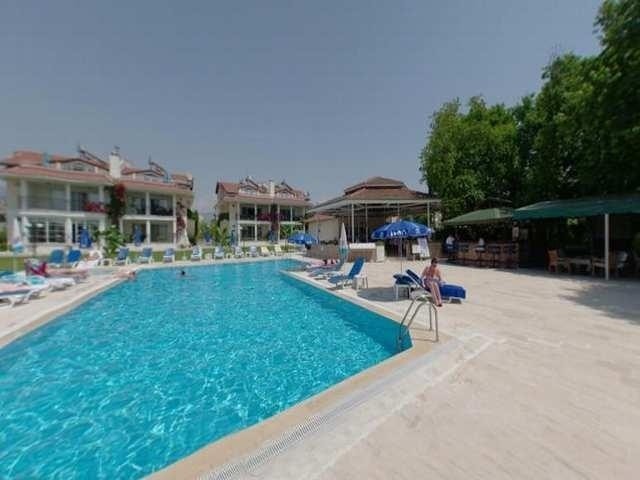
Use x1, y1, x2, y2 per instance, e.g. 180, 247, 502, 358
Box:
2, 248, 84, 310
453, 242, 629, 277
89, 243, 298, 262
308, 245, 466, 308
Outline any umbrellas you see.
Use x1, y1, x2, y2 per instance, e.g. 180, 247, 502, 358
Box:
339, 222, 349, 273
287, 231, 319, 258
371, 216, 433, 273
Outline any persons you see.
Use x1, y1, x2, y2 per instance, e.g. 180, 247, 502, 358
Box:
475, 235, 485, 252
307, 257, 335, 270
127, 271, 137, 281
420, 257, 443, 307
446, 234, 455, 261
